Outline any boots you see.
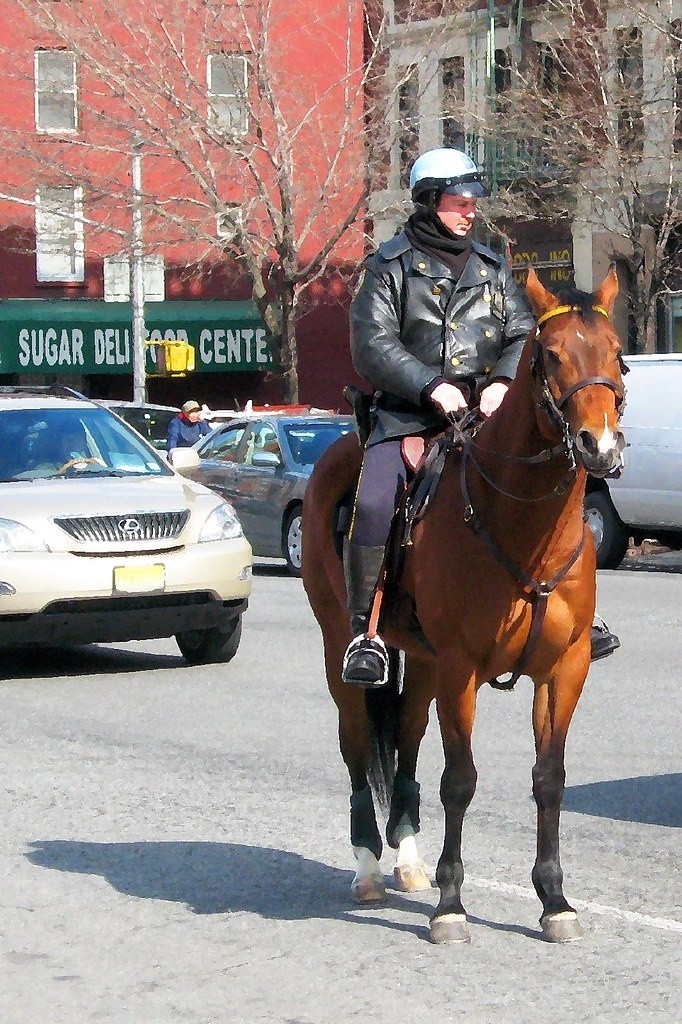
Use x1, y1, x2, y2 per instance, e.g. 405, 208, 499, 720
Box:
342, 536, 385, 681
589, 626, 621, 658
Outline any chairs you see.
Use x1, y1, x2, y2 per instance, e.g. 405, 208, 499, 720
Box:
312, 432, 337, 454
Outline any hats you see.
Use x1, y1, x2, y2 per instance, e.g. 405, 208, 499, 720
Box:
181, 401, 202, 414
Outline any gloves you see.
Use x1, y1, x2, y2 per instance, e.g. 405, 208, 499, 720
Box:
479, 380, 509, 417
429, 380, 468, 412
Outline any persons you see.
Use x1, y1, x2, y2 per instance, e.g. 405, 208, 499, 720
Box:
343, 149, 621, 687
167, 402, 215, 459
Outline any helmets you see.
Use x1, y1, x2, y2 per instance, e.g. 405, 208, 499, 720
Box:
410, 147, 488, 199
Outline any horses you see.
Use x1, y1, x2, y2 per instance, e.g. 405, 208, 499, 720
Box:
301, 257, 628, 948
